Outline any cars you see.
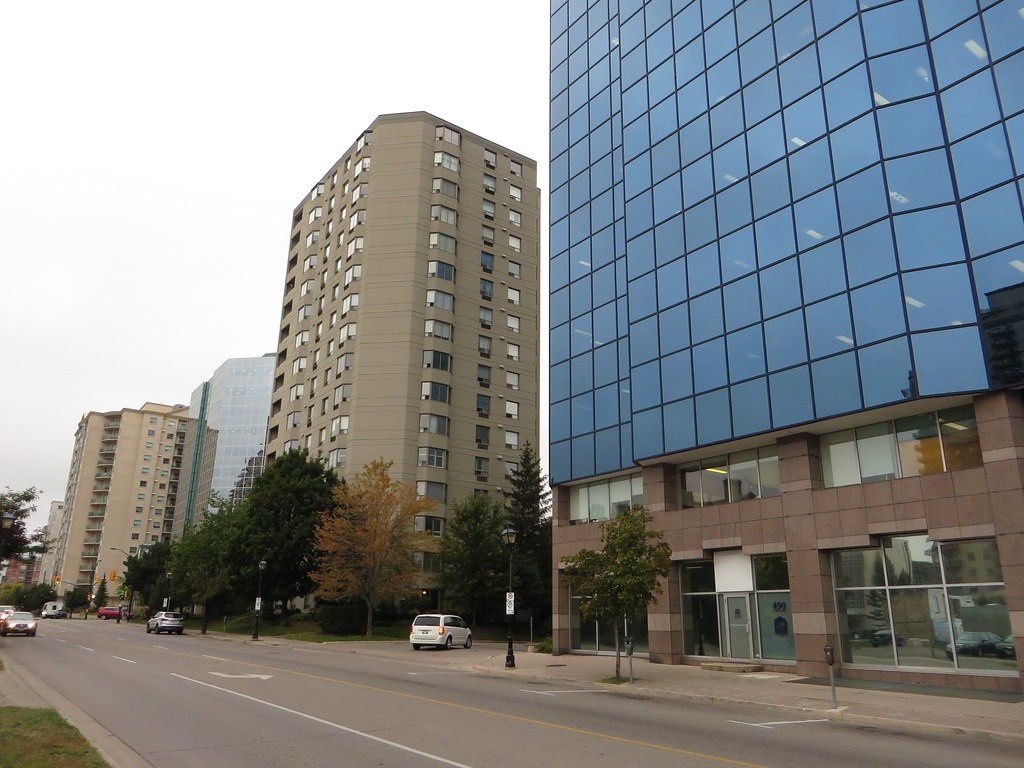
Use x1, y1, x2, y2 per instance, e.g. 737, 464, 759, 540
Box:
947, 630, 1003, 662
870, 630, 905, 648
0, 611, 39, 636
0, 610, 13, 623
996, 635, 1016, 659
54, 609, 67, 619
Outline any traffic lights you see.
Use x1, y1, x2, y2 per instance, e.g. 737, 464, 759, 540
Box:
124, 590, 128, 597
55, 576, 61, 582
89, 595, 92, 601
95, 575, 99, 583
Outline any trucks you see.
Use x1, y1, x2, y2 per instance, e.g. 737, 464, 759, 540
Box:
41, 602, 62, 618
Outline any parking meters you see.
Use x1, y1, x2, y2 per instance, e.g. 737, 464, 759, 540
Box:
624, 637, 635, 683
824, 645, 838, 710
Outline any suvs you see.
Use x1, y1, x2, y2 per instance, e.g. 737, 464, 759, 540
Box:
409, 613, 473, 651
98, 606, 132, 621
146, 611, 185, 634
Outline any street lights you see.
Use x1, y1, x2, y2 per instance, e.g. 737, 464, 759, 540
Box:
502, 525, 518, 668
110, 547, 136, 622
251, 560, 267, 640
